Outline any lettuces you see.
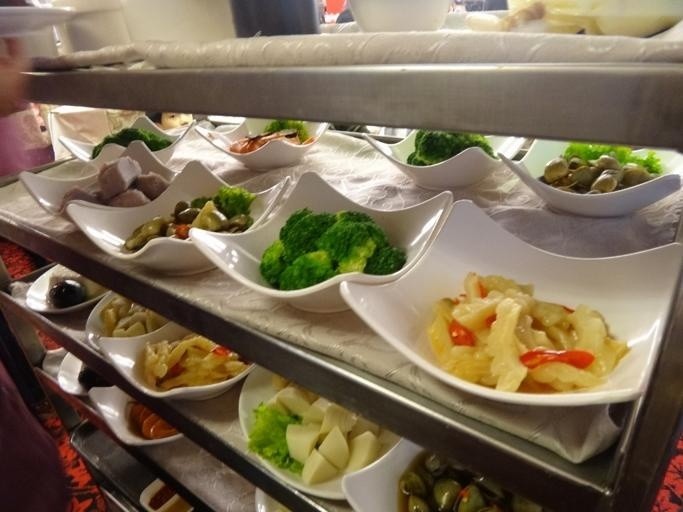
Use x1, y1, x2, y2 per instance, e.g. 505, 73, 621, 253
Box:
248, 402, 304, 477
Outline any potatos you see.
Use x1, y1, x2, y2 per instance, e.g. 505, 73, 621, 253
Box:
99, 296, 170, 337
265, 374, 402, 486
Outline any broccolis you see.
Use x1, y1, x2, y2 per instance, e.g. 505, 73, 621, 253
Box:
92, 127, 172, 158
405, 128, 498, 165
259, 206, 407, 290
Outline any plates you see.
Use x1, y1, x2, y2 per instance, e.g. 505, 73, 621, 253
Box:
497, 136, 683, 215
58, 158, 290, 282
0, 6, 78, 39
341, 195, 680, 415
54, 116, 196, 166
26, 265, 683, 512
193, 115, 333, 161
182, 172, 454, 319
363, 121, 529, 190
19, 141, 179, 228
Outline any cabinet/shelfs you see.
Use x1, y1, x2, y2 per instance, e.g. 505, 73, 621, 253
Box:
1, 65, 682, 512
